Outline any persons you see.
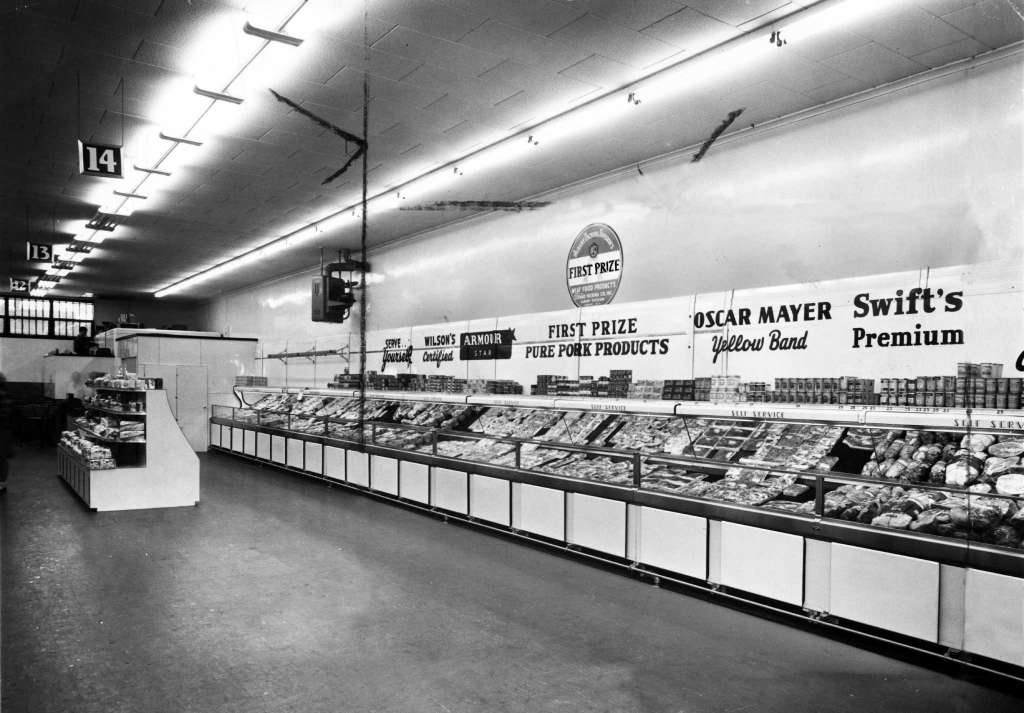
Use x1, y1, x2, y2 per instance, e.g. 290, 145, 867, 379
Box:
73, 327, 90, 354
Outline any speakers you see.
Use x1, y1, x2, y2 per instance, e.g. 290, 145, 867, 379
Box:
310, 276, 345, 323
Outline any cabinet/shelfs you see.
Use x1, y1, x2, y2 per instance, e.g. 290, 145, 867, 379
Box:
57, 388, 199, 512
